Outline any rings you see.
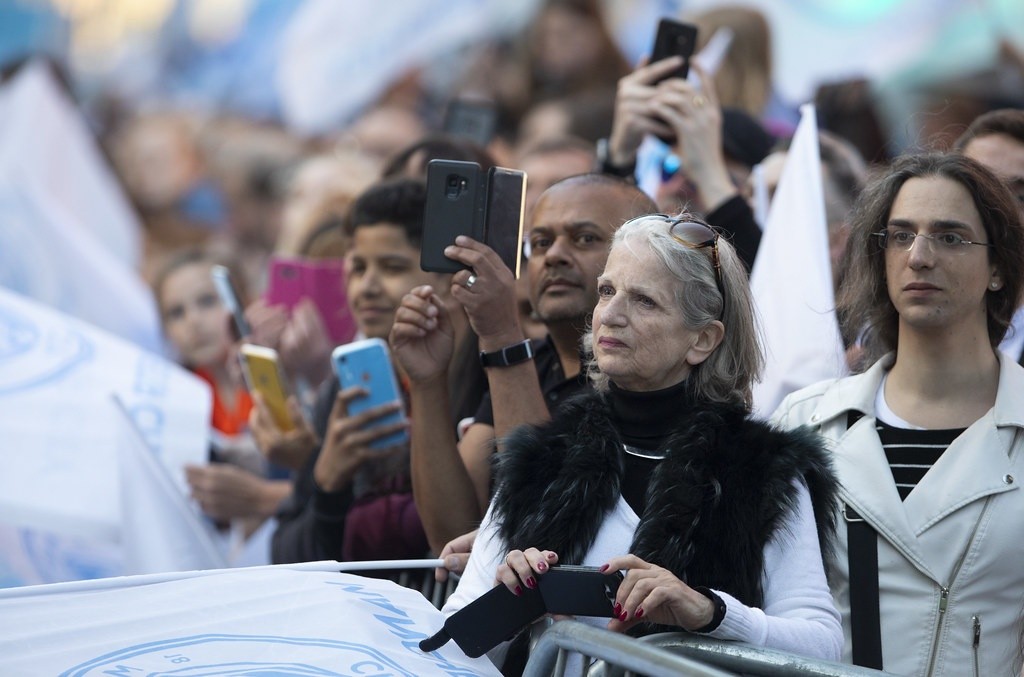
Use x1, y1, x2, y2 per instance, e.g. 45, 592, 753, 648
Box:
466, 274, 477, 289
693, 94, 707, 108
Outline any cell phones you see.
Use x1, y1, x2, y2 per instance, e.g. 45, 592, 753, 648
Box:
260, 259, 309, 322
419, 157, 480, 281
237, 343, 299, 438
330, 336, 411, 454
532, 562, 625, 619
645, 18, 702, 148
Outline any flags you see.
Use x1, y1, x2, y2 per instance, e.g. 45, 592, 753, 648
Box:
287, 1, 487, 133
0, 70, 274, 585
0, 571, 502, 677
744, 110, 848, 424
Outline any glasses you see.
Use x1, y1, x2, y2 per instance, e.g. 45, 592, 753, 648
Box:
877, 226, 998, 256
659, 154, 685, 184
626, 213, 726, 325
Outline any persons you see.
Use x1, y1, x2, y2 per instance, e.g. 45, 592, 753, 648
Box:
103, 0, 904, 609
440, 213, 843, 677
766, 151, 1024, 677
956, 109, 1024, 369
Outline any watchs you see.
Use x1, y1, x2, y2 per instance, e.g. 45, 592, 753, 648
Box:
596, 139, 637, 178
478, 338, 535, 367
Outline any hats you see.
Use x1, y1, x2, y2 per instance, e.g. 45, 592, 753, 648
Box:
721, 107, 775, 168
